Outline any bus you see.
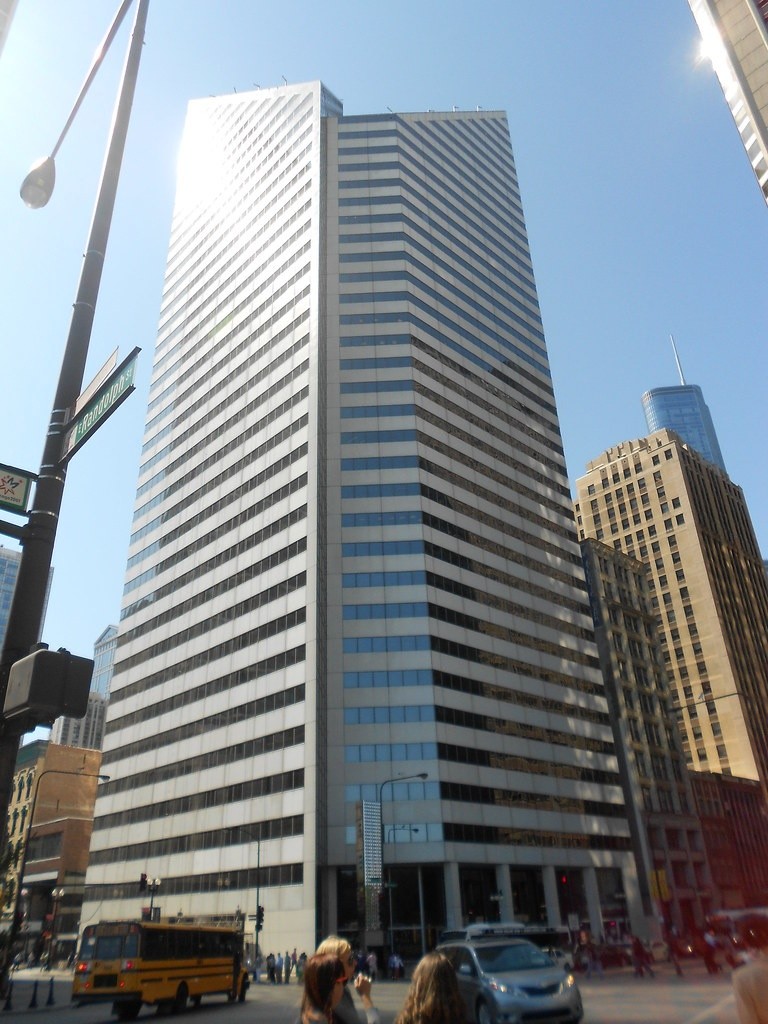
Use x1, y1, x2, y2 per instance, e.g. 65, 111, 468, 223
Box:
705, 908, 768, 950
71, 918, 249, 1018
438, 924, 575, 969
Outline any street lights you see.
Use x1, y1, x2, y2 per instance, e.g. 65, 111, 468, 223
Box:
0, 0, 150, 844
45, 888, 65, 968
379, 773, 428, 973
146, 878, 161, 920
387, 827, 419, 844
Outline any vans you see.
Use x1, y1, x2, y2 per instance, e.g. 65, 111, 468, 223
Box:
433, 939, 584, 1024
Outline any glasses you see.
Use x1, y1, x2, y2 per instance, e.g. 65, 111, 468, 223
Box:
336, 977, 349, 987
343, 951, 356, 967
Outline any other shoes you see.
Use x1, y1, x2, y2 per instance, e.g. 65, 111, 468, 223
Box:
254, 979, 305, 986
676, 970, 686, 977
633, 973, 645, 978
588, 975, 595, 981
650, 972, 657, 977
600, 976, 606, 981
392, 976, 400, 980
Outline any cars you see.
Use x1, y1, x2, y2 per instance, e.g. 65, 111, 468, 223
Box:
642, 940, 670, 964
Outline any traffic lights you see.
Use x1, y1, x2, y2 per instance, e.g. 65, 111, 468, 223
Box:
223, 827, 259, 981
257, 906, 264, 923
140, 873, 147, 891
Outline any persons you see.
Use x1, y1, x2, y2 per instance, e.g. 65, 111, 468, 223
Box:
244, 930, 763, 977
394, 953, 470, 1024
299, 954, 349, 1024
11, 951, 74, 972
729, 927, 768, 1024
315, 937, 381, 1024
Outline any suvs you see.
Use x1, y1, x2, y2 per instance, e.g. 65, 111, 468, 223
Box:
603, 944, 650, 967
